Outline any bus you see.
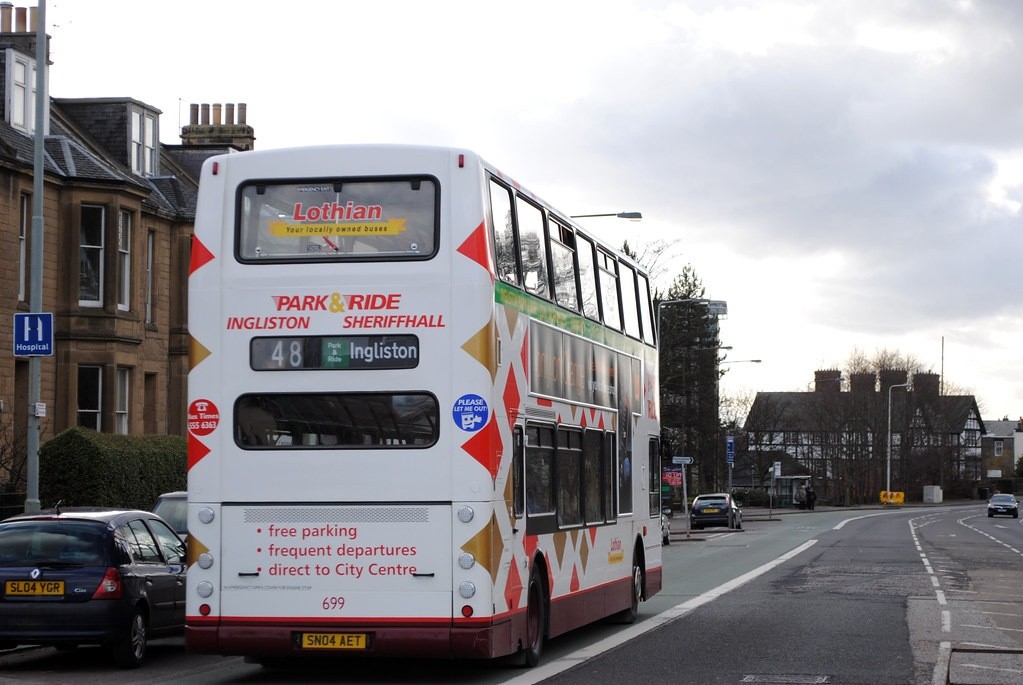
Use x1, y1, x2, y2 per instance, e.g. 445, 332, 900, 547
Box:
182, 139, 667, 672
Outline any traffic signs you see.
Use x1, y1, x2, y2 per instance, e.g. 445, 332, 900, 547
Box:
672, 456, 695, 465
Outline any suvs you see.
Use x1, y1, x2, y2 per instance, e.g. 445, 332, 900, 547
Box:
148, 489, 188, 549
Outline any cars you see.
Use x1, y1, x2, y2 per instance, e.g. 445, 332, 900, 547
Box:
985, 493, 1020, 519
659, 495, 682, 544
689, 492, 743, 531
0, 506, 189, 670
733, 489, 766, 509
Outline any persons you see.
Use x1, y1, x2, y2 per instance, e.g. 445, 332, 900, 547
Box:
798, 484, 816, 510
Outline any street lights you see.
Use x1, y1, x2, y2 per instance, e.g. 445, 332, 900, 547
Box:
886, 383, 913, 499
715, 359, 762, 492
657, 298, 728, 334
685, 346, 733, 486
